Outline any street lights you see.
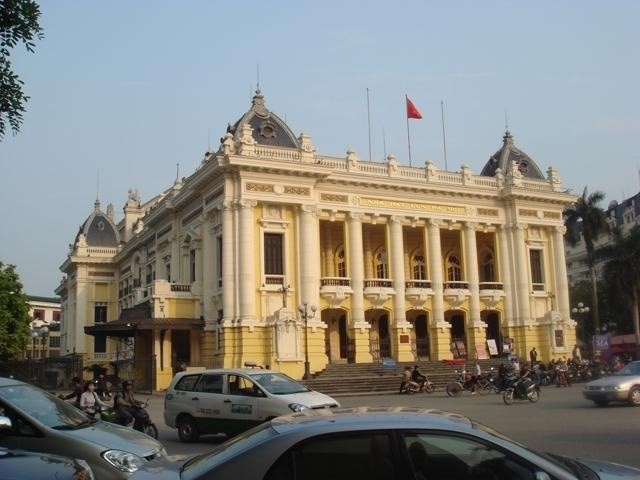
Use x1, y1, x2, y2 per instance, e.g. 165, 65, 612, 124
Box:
32, 330, 38, 378
298, 300, 318, 381
40, 326, 50, 387
572, 301, 590, 344
596, 321, 617, 337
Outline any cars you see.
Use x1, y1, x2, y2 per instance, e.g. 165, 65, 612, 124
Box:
124, 408, 639, 480
582, 360, 640, 407
0, 447, 95, 479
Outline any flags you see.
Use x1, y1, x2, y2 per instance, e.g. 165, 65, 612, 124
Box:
405, 95, 422, 119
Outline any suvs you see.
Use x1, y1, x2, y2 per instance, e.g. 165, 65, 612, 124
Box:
0, 377, 169, 479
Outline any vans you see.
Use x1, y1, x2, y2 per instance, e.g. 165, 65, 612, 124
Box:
164, 361, 341, 441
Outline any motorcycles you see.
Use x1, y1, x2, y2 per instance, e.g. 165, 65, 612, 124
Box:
405, 375, 435, 395
447, 360, 596, 397
503, 380, 541, 404
121, 397, 157, 440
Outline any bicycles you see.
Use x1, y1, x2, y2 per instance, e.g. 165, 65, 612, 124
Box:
85, 404, 120, 423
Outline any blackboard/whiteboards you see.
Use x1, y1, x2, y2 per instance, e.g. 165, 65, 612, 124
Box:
486, 339, 499, 355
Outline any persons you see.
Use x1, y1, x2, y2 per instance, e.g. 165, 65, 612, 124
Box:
393, 337, 632, 396
59, 368, 141, 426
